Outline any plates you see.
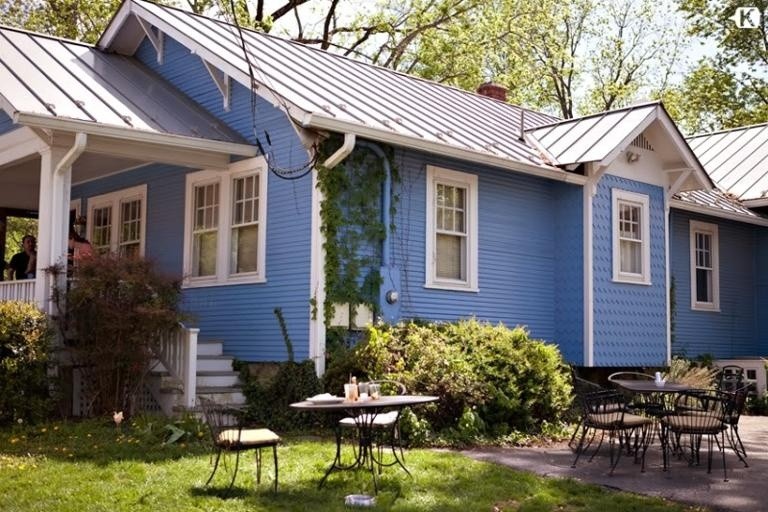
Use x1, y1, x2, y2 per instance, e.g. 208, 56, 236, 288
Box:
306, 398, 345, 404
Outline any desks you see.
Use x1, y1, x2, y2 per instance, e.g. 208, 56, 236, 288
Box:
289, 395, 440, 496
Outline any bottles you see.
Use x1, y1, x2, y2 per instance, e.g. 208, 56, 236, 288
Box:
655, 372, 662, 386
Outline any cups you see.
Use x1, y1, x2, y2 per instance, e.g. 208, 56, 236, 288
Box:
344, 382, 381, 402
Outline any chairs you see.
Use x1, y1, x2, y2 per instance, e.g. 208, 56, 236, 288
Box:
198, 396, 283, 500
335, 380, 405, 474
568, 364, 754, 482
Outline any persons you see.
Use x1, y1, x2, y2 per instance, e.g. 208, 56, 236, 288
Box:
6, 234, 36, 281
65, 231, 101, 307
